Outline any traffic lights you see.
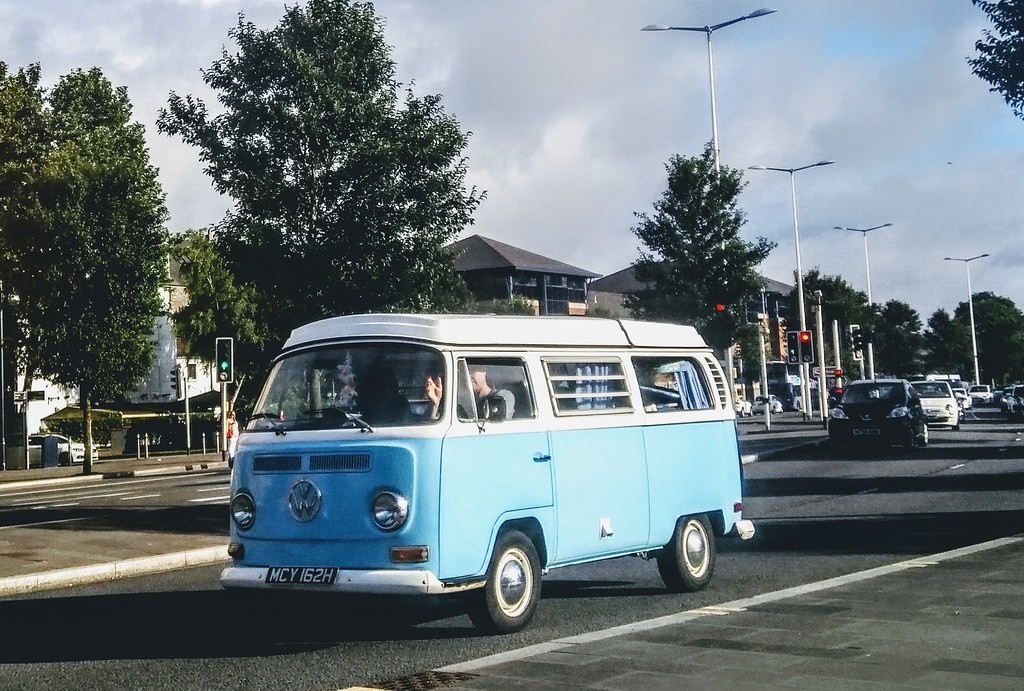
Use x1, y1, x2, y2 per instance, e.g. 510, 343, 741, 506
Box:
800, 330, 814, 364
787, 331, 801, 365
850, 325, 864, 360
717, 306, 726, 311
216, 337, 234, 383
834, 369, 842, 377
170, 367, 184, 401
836, 388, 843, 395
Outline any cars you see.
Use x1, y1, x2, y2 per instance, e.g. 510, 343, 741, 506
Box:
751, 395, 785, 416
28, 432, 99, 466
910, 381, 963, 430
901, 374, 1024, 423
827, 379, 928, 453
734, 395, 753, 417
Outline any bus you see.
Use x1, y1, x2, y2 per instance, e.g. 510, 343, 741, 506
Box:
759, 361, 819, 411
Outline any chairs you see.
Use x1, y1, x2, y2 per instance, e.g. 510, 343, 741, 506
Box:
853, 389, 870, 402
356, 367, 410, 420
887, 387, 901, 400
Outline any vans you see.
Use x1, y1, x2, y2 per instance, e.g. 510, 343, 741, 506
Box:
217, 314, 763, 635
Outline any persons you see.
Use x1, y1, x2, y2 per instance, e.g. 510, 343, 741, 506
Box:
420, 361, 515, 419
228, 410, 239, 470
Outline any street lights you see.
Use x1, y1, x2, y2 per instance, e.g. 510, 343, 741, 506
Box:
942, 253, 991, 387
834, 222, 896, 379
748, 160, 835, 420
639, 5, 779, 399
